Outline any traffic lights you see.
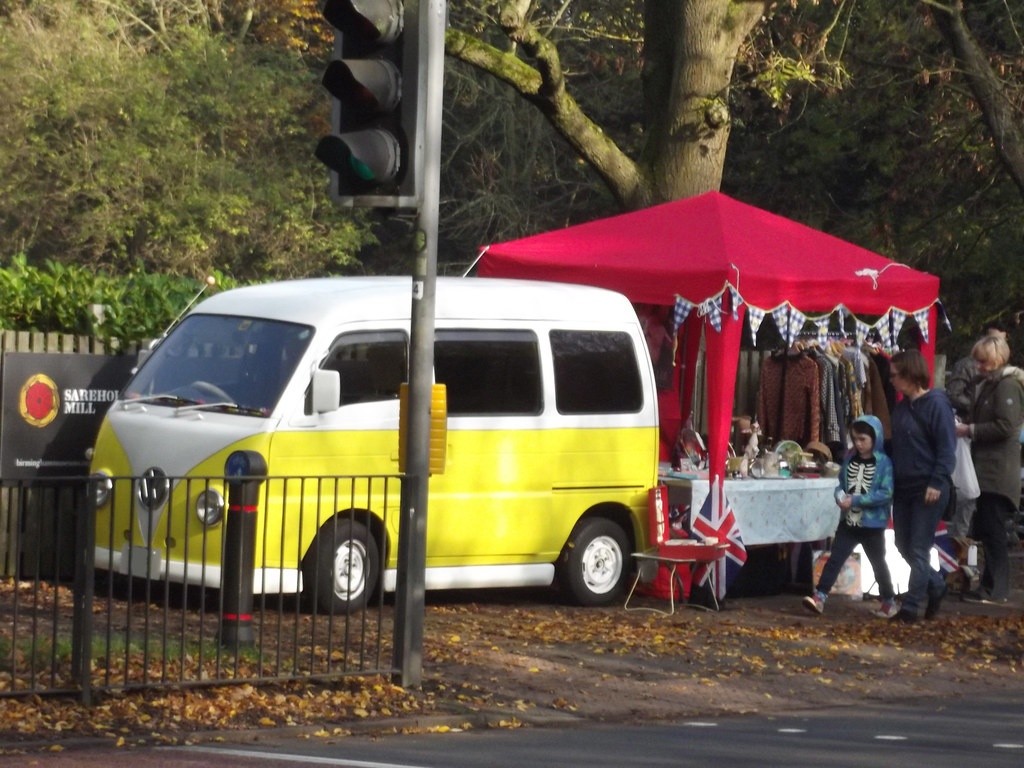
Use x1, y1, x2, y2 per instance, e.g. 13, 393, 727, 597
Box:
314, 0, 424, 209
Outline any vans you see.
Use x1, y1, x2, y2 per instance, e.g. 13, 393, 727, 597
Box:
86, 276, 662, 614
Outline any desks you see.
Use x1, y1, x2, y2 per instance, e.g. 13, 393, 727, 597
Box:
658, 475, 841, 554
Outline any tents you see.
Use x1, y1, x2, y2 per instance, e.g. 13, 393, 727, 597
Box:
474, 189, 940, 600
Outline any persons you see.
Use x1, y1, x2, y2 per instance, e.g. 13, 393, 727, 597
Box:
951, 321, 1011, 423
886, 349, 959, 620
955, 335, 1024, 605
802, 413, 900, 619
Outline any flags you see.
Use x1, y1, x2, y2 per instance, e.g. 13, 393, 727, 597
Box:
687, 473, 747, 601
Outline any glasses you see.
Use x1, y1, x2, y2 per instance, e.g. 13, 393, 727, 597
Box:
888, 372, 903, 378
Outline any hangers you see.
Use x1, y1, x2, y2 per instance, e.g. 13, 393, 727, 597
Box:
773, 330, 882, 349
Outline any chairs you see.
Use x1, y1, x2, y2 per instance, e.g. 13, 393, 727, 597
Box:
624, 486, 727, 617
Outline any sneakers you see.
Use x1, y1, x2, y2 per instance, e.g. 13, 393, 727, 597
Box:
960, 587, 1014, 606
802, 587, 949, 623
